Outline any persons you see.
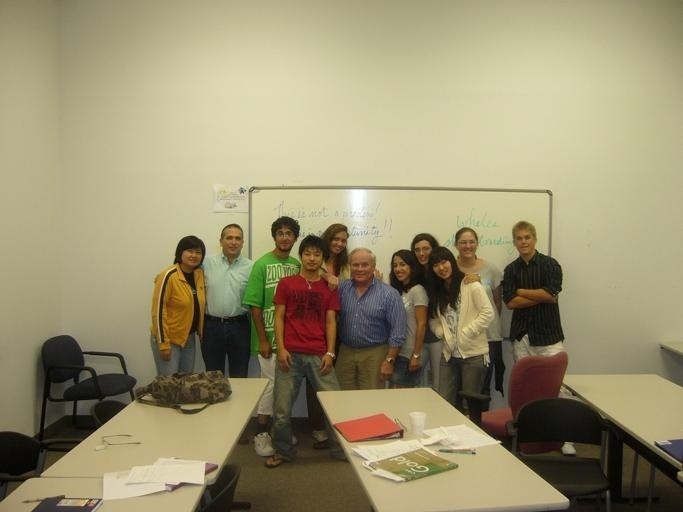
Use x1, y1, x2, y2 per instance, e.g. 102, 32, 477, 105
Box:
429, 245, 495, 428
410, 232, 444, 395
453, 226, 505, 403
301, 223, 348, 444
501, 220, 578, 459
373, 249, 430, 388
241, 216, 302, 457
147, 234, 206, 379
200, 222, 255, 445
320, 247, 407, 462
263, 232, 341, 469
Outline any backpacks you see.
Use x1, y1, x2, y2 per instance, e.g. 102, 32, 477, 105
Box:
135, 369, 231, 414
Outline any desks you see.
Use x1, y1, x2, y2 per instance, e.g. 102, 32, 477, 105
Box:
0, 378, 270, 512
314, 388, 570, 512
562, 374, 683, 512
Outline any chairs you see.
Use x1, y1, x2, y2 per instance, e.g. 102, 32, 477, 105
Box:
0, 335, 136, 512
507, 397, 610, 512
480, 350, 569, 453
197, 464, 252, 512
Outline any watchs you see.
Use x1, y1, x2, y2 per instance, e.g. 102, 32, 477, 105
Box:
384, 357, 395, 366
411, 351, 422, 361
325, 351, 336, 360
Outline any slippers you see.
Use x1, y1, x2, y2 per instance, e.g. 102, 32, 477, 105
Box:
265, 455, 286, 468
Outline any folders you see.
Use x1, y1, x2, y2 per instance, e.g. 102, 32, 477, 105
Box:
333, 413, 403, 442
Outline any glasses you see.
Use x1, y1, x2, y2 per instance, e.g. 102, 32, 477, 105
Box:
102, 434, 140, 447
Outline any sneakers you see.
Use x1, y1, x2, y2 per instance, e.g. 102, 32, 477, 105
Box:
561, 443, 576, 456
254, 433, 276, 456
313, 430, 328, 442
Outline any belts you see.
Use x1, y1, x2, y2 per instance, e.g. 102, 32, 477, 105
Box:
204, 315, 248, 323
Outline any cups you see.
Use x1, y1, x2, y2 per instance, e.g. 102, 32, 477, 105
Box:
409, 412, 426, 436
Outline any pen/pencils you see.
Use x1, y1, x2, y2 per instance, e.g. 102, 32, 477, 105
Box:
438, 449, 476, 454
22, 495, 44, 504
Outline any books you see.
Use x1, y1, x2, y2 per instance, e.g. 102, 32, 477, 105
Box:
163, 461, 218, 493
362, 447, 458, 483
31, 496, 103, 512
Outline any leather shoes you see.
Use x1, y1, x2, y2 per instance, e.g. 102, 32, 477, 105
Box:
314, 439, 339, 450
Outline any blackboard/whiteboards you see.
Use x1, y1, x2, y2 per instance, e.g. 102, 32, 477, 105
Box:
248, 187, 553, 341
